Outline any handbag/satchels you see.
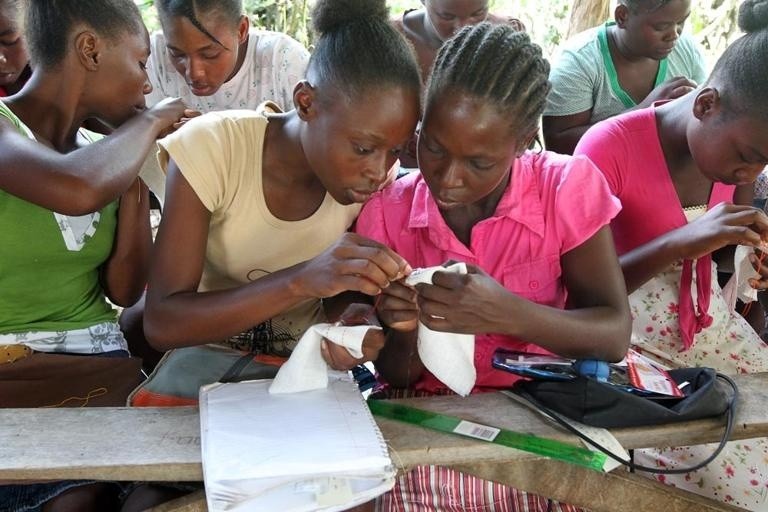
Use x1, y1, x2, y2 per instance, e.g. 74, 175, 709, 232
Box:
514, 367, 732, 429
125, 343, 290, 406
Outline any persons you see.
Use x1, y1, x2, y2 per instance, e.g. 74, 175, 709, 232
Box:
391, 0, 526, 86
571, 0, 768, 512
542, 0, 708, 159
0, 0, 188, 512
120, 0, 422, 512
119, 0, 312, 357
0, 0, 34, 99
356, 21, 633, 512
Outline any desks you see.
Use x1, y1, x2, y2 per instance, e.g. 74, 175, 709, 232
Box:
0, 371, 768, 511
